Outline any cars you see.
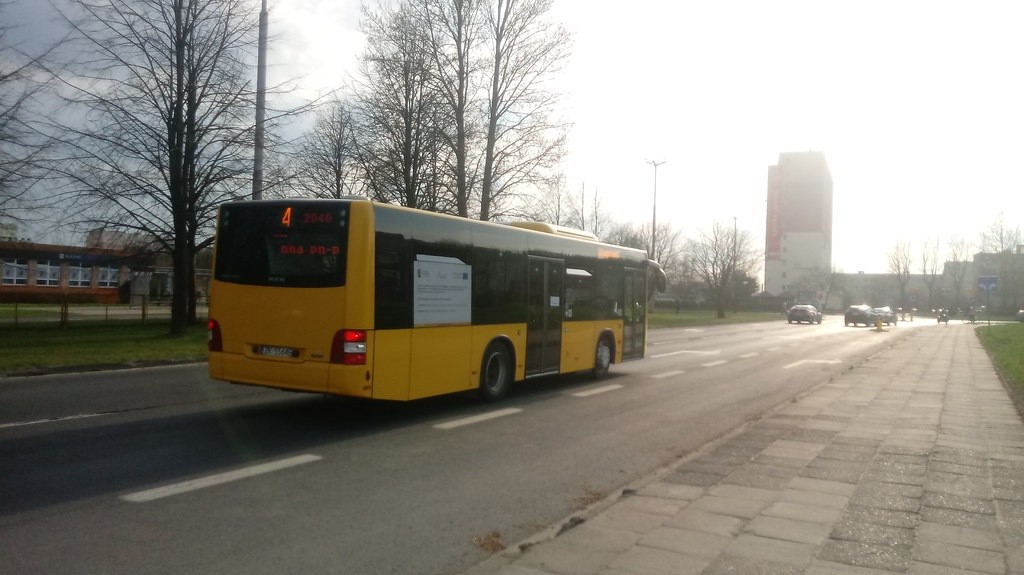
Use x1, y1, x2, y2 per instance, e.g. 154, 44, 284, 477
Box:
787, 304, 822, 324
874, 307, 898, 325
845, 304, 879, 327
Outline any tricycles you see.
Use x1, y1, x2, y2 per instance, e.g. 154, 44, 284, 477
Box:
937, 313, 949, 324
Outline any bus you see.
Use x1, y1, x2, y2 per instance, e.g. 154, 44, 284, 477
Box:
207, 194, 668, 402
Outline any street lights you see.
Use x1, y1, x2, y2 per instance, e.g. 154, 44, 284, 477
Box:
646, 158, 666, 259
